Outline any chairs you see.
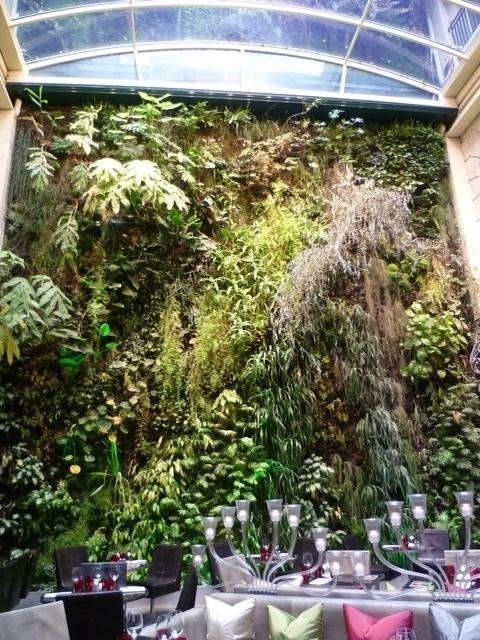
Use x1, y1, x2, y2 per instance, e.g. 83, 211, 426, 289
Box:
205, 528, 480, 601
1, 549, 38, 610
128, 545, 183, 628
54, 591, 124, 638
54, 546, 88, 591
2, 601, 69, 639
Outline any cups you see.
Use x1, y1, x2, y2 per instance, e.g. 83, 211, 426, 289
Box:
109, 565, 121, 584
167, 610, 185, 640
92, 566, 103, 584
156, 613, 173, 640
302, 552, 313, 568
71, 565, 84, 585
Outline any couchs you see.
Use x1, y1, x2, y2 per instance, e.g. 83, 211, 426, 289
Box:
139, 592, 479, 640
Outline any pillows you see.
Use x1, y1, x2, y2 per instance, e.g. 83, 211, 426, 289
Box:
267, 603, 324, 639
342, 603, 412, 639
429, 603, 480, 639
204, 598, 255, 639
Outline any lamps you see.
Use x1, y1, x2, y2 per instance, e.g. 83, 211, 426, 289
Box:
347, 492, 480, 604
190, 499, 343, 597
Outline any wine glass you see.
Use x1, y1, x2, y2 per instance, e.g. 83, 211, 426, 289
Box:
126, 608, 144, 640
